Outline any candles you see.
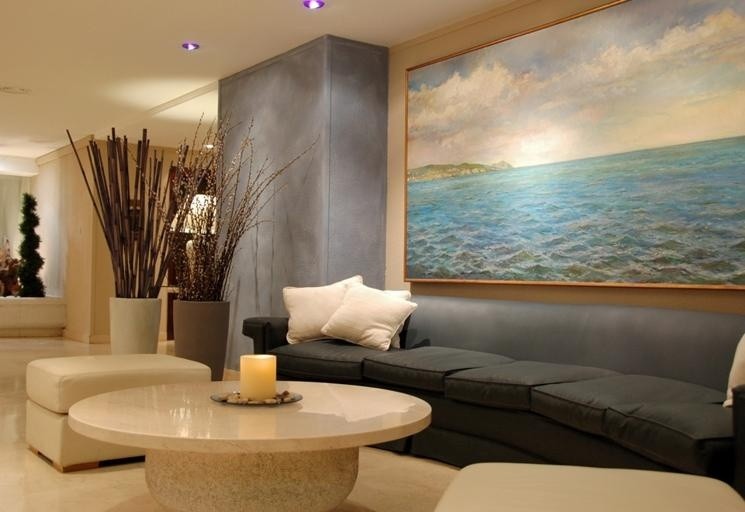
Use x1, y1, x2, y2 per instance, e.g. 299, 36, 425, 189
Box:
238, 352, 278, 401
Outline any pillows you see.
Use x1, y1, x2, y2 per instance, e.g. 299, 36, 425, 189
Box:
282, 275, 417, 353
722, 331, 745, 408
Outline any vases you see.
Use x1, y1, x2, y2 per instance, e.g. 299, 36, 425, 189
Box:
110, 296, 230, 381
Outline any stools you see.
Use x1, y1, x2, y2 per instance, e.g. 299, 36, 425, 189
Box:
434, 458, 745, 512
22, 352, 212, 475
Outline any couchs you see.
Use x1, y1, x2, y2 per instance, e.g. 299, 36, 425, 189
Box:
241, 295, 745, 496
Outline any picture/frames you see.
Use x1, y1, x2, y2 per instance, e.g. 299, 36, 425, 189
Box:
400, 1, 743, 288
